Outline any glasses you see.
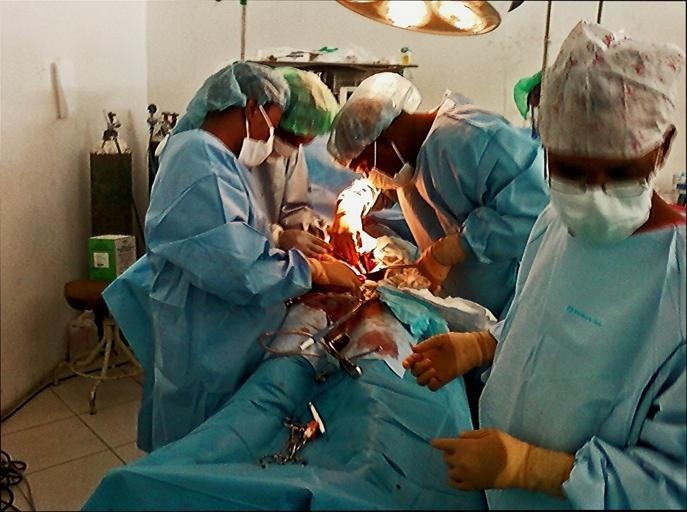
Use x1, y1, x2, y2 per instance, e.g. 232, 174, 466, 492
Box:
543, 145, 660, 198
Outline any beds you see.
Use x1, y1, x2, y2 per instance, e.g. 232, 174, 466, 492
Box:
77, 133, 488, 511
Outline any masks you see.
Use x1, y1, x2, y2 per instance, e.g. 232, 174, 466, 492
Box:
547, 176, 655, 245
236, 104, 276, 169
366, 138, 414, 190
272, 137, 300, 159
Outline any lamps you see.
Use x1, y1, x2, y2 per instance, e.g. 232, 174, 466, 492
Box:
336, 0, 526, 37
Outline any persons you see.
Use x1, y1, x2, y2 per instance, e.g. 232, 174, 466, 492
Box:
329, 71, 551, 321
101, 58, 363, 456
402, 20, 686, 512
245, 63, 332, 257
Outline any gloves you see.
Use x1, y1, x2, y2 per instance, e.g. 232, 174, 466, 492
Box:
412, 230, 465, 289
307, 253, 361, 291
324, 202, 365, 265
279, 225, 333, 261
427, 427, 574, 499
401, 329, 496, 392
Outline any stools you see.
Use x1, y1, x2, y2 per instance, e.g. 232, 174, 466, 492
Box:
51, 277, 145, 416
